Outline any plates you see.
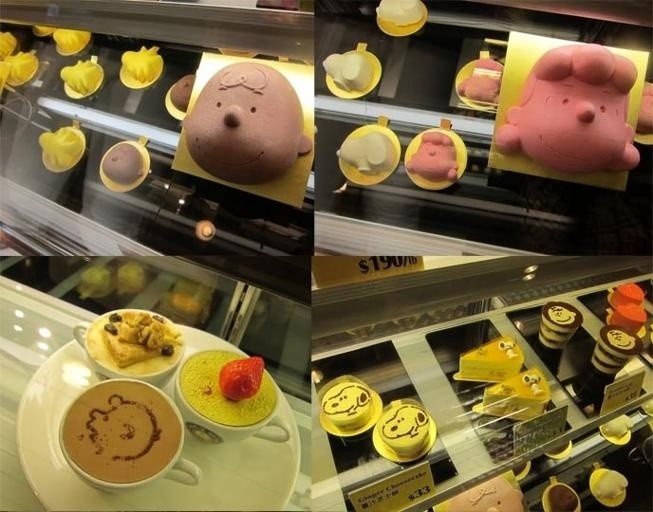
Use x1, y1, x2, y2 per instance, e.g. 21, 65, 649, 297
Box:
15, 321, 303, 508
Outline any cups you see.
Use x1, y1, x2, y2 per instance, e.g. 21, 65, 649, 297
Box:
376, 397, 430, 460
178, 347, 291, 455
57, 377, 202, 497
603, 277, 648, 334
72, 309, 186, 387
589, 326, 643, 377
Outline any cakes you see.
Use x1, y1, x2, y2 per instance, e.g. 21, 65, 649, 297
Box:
0, 24, 196, 186
459, 336, 524, 382
379, 405, 431, 459
591, 323, 642, 374
181, 62, 313, 186
449, 476, 522, 512
635, 82, 653, 135
61, 308, 277, 486
494, 43, 640, 173
481, 367, 550, 420
477, 400, 653, 512
321, 1, 505, 183
609, 282, 647, 334
